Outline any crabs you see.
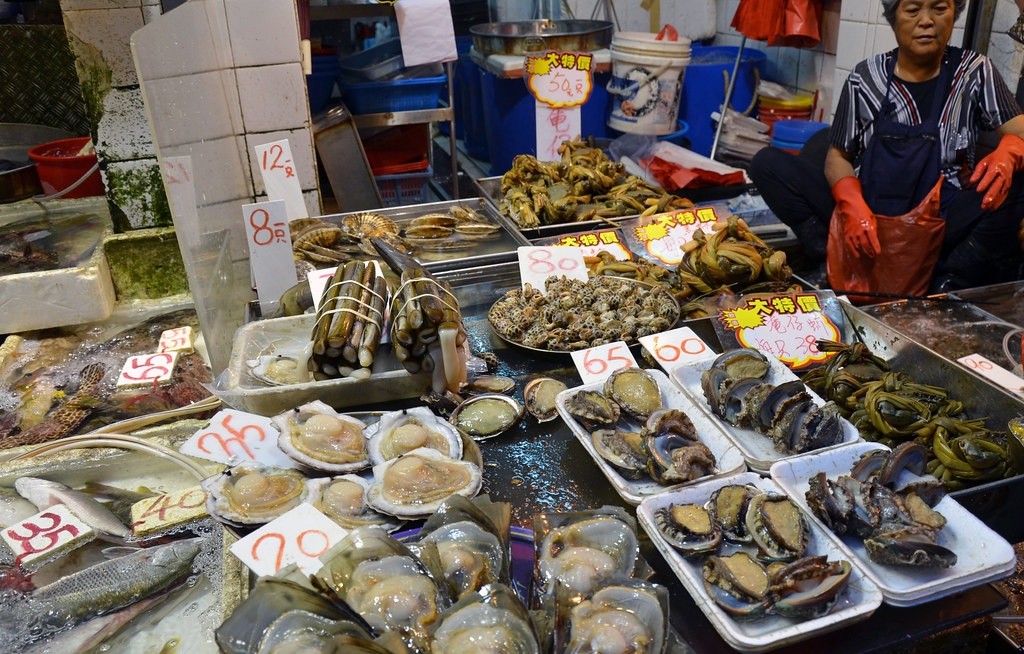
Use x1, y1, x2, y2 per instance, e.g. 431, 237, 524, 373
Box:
582, 216, 794, 322
501, 147, 693, 229
803, 337, 1023, 493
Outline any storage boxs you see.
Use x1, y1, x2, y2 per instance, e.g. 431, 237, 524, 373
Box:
1, 15, 834, 336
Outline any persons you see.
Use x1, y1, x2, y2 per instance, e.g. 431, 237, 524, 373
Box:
749, 0, 1023, 293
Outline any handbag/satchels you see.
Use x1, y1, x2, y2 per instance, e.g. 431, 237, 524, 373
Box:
824, 178, 947, 298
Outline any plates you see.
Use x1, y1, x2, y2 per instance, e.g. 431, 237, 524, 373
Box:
667, 347, 863, 478
487, 274, 681, 358
553, 369, 749, 508
250, 195, 535, 290
771, 441, 1021, 608
634, 468, 884, 649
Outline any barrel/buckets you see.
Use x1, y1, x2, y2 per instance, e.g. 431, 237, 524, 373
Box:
434, 33, 832, 173
28, 136, 106, 200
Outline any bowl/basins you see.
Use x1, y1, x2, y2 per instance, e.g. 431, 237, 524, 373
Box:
469, 19, 614, 56
0, 123, 79, 204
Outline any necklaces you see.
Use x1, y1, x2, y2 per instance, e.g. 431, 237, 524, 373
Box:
896, 54, 899, 80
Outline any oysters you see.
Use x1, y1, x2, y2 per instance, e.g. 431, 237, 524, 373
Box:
215, 493, 670, 654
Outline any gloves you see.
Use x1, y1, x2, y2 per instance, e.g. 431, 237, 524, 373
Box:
831, 172, 881, 258
972, 135, 1023, 208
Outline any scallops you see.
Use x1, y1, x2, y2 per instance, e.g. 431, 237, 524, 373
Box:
287, 202, 501, 288
208, 397, 484, 537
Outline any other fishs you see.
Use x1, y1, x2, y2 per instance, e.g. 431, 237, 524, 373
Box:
0, 212, 104, 277
0, 307, 214, 452
0, 475, 224, 644
0, 153, 35, 173
